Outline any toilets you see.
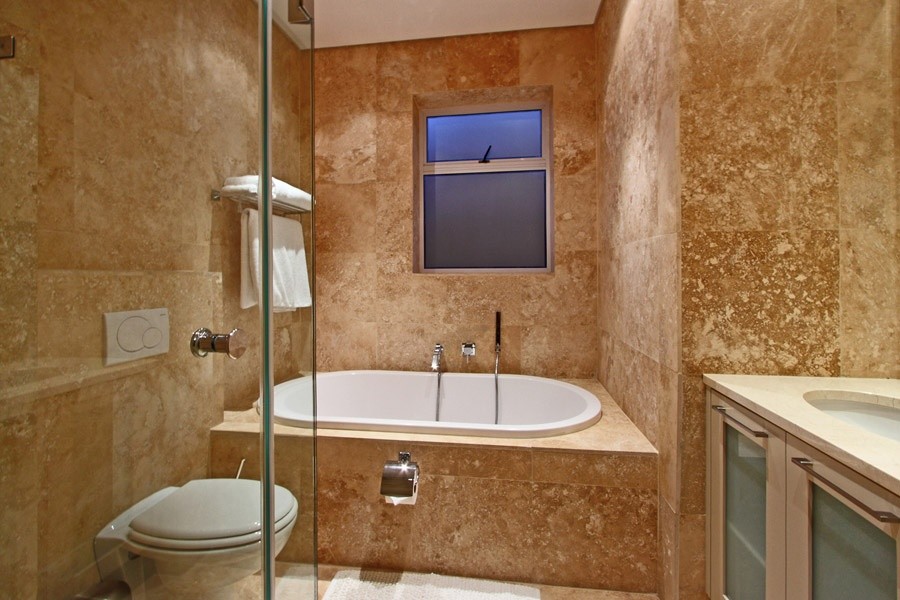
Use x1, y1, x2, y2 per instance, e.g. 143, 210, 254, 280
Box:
93, 477, 299, 600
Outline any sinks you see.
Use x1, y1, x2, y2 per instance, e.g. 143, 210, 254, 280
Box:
802, 389, 899, 444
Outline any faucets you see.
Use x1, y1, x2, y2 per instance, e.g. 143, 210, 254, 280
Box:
431, 344, 444, 372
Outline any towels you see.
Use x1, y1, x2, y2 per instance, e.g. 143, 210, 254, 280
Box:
219, 175, 317, 211
239, 207, 313, 314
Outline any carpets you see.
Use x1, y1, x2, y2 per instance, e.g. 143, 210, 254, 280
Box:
322, 570, 541, 600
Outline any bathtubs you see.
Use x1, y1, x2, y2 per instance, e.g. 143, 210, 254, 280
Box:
253, 369, 602, 439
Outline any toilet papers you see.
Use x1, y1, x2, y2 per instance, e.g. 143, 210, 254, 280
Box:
384, 470, 420, 506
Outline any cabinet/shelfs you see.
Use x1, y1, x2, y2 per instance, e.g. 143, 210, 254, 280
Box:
707, 387, 900, 600
